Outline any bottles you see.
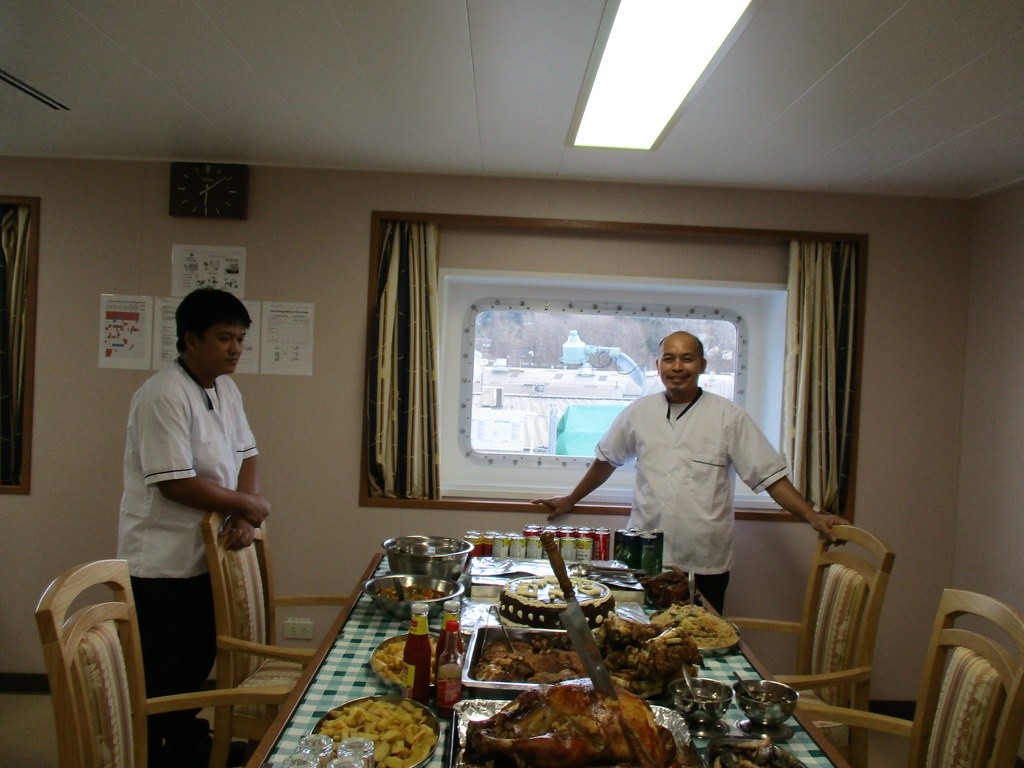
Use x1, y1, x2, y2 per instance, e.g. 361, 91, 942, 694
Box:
404, 602, 431, 709
434, 601, 465, 719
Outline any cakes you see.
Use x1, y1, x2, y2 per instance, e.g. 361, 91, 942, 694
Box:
497, 574, 615, 632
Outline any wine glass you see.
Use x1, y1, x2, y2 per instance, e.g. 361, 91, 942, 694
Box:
669, 679, 735, 739
733, 679, 799, 740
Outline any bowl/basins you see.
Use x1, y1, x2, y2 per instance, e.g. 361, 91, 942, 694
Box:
360, 574, 465, 622
382, 536, 476, 580
704, 735, 808, 768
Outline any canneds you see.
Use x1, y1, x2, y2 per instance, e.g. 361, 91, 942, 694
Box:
463, 525, 611, 562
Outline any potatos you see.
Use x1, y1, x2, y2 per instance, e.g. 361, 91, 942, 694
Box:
315, 698, 437, 768
372, 636, 440, 686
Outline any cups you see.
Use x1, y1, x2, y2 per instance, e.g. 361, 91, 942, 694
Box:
272, 733, 375, 768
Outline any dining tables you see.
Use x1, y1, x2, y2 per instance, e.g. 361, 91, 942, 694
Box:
245, 553, 850, 767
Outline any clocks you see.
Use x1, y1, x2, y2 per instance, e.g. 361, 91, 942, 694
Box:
169, 162, 248, 221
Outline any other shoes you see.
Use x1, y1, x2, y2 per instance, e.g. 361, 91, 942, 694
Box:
164, 718, 247, 766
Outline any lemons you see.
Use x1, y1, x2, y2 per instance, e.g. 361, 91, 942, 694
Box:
682, 618, 698, 630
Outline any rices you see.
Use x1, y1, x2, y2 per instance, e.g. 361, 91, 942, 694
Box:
650, 603, 740, 649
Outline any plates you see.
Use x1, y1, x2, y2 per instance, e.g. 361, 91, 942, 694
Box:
369, 633, 441, 692
310, 695, 439, 768
650, 611, 741, 655
588, 559, 628, 575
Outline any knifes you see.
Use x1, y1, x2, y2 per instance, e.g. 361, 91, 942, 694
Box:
539, 530, 616, 700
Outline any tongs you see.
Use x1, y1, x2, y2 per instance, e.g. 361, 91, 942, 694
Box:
478, 605, 518, 660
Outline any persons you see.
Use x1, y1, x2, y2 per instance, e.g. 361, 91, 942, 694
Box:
532, 331, 850, 617
118, 286, 272, 768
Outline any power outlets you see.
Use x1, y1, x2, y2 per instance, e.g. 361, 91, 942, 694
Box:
283, 621, 313, 639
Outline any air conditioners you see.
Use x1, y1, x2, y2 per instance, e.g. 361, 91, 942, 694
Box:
481, 387, 503, 407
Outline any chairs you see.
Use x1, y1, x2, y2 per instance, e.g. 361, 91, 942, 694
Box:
201, 508, 349, 768
796, 589, 1024, 768
722, 524, 896, 768
34, 559, 291, 768
